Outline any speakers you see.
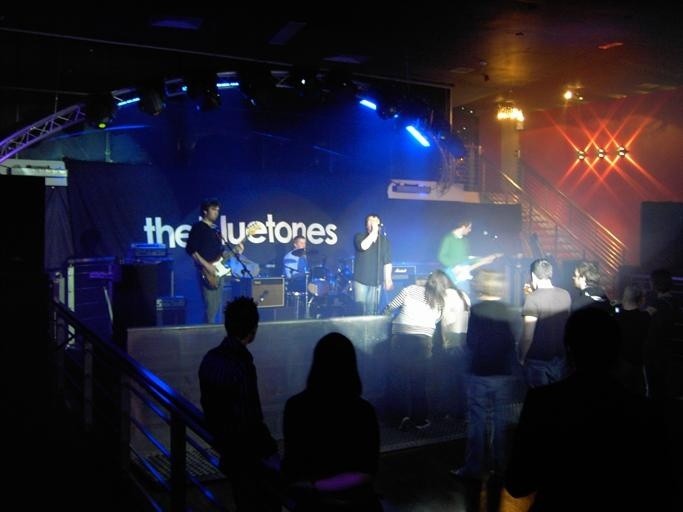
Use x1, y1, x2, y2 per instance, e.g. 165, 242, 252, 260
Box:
0, 175, 74, 269
641, 201, 683, 278
116, 263, 171, 340
72, 264, 110, 344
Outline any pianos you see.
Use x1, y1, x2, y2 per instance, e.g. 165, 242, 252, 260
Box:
340, 265, 416, 280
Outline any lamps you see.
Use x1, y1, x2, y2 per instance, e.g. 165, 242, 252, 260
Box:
496, 89, 524, 121
577, 147, 627, 160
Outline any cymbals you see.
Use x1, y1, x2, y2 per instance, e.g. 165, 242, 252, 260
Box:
291, 247, 315, 258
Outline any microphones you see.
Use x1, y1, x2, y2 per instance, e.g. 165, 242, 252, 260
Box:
483, 230, 498, 239
378, 224, 383, 226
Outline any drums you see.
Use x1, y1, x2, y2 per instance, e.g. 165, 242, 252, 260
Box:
309, 290, 346, 319
308, 265, 332, 295
291, 273, 311, 296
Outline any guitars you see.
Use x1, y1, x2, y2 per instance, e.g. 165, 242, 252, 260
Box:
202, 222, 261, 290
451, 254, 504, 284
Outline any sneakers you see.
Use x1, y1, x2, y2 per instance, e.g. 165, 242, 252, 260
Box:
397, 415, 431, 432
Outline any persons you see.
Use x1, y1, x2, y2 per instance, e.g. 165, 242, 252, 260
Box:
282, 234, 310, 283
278, 332, 382, 510
195, 294, 283, 510
384, 215, 682, 512
350, 214, 394, 316
185, 197, 244, 325
